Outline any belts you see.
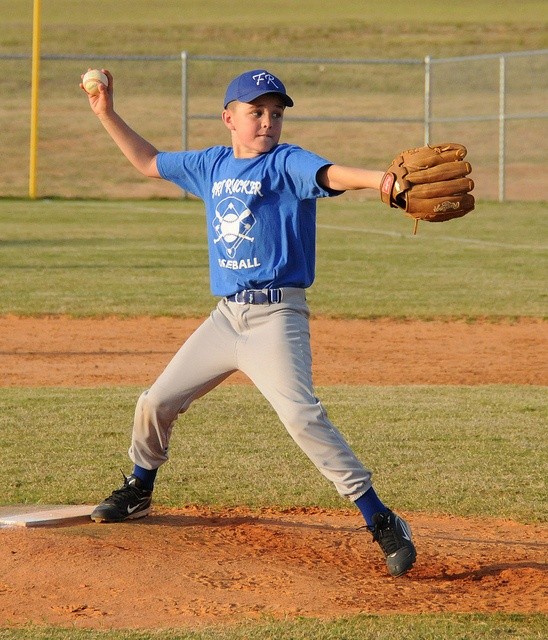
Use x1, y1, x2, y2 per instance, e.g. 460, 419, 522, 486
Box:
228, 288, 280, 304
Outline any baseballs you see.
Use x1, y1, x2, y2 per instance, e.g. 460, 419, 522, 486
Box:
82, 69, 109, 98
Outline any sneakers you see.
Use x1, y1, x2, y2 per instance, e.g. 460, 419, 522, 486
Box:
90, 469, 154, 523
355, 510, 417, 577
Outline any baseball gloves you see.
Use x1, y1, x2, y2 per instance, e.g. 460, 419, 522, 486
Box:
379, 143, 475, 235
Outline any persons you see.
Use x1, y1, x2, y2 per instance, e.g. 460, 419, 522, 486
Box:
78, 66, 476, 578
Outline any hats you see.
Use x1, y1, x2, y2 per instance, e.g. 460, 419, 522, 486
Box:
223, 69, 294, 109
